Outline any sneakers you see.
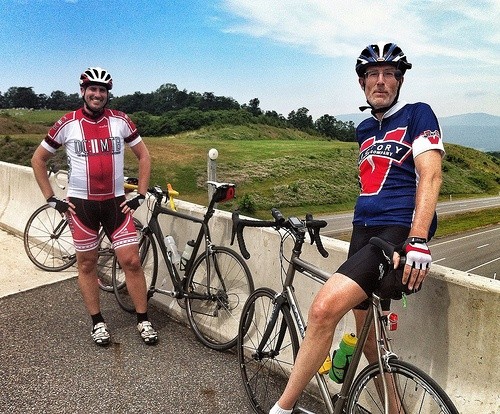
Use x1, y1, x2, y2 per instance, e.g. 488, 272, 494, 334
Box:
91, 322, 111, 345
137, 321, 159, 345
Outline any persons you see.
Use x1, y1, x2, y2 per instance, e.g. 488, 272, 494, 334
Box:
270, 42, 446, 414
31, 68, 158, 345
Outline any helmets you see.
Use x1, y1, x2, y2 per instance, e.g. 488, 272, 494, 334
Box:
79, 67, 112, 90
356, 43, 412, 75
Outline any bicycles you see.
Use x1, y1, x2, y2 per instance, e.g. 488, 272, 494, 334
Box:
227, 205, 460, 414
112, 180, 258, 351
22, 159, 149, 293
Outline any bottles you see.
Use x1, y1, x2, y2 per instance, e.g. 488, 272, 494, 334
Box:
164, 234, 181, 264
304, 325, 332, 376
179, 240, 196, 267
330, 332, 358, 384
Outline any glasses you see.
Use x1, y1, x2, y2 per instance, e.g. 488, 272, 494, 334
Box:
364, 71, 394, 79
